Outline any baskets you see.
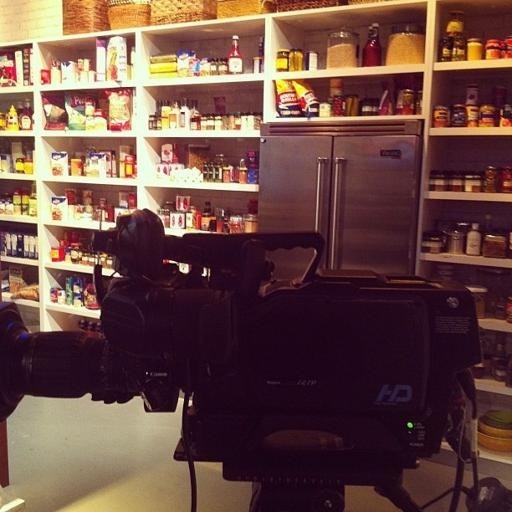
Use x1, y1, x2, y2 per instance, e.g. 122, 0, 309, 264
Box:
108, 3, 152, 29
153, 0, 218, 25
219, 0, 269, 18
276, 0, 343, 15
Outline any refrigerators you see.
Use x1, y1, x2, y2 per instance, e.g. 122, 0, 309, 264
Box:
256, 119, 423, 280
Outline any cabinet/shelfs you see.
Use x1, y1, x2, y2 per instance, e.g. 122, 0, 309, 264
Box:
0, 13, 266, 336
413, 2, 512, 463
263, 1, 431, 124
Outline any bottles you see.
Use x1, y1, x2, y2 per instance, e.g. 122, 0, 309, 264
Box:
19, 99, 34, 130
463, 223, 481, 255
7, 105, 20, 131
228, 35, 243, 73
200, 59, 228, 75
156, 98, 198, 129
239, 150, 259, 184
201, 202, 212, 231
362, 23, 382, 67
330, 80, 344, 118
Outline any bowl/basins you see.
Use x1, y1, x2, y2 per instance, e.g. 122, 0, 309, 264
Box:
474, 409, 512, 453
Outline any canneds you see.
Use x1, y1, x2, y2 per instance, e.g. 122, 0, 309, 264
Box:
252, 57, 264, 72
276, 49, 318, 70
190, 112, 242, 130
343, 94, 359, 116
397, 88, 423, 115
16, 156, 26, 173
428, 165, 512, 194
203, 153, 233, 181
433, 84, 511, 127
79, 319, 103, 339
438, 10, 512, 62
158, 200, 174, 227
